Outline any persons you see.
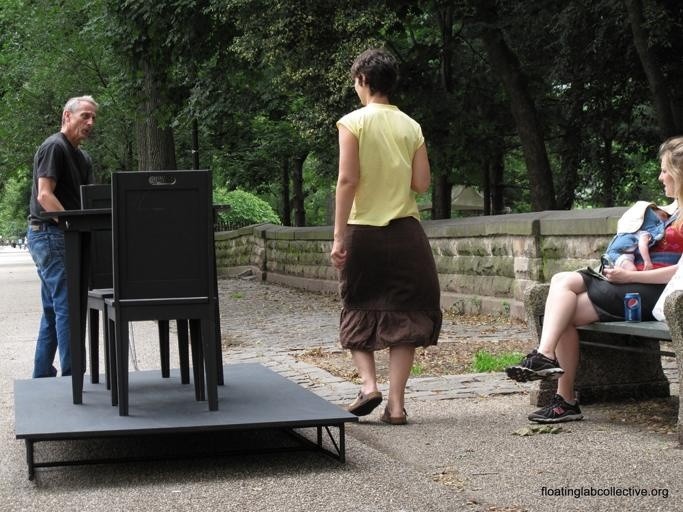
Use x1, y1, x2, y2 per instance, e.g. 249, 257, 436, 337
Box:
504, 136, 683, 423
27, 95, 100, 379
329, 48, 442, 422
603, 208, 668, 272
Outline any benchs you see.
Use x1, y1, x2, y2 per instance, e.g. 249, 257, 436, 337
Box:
524, 283, 683, 449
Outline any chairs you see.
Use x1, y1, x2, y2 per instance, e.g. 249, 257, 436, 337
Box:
80, 184, 189, 391
104, 170, 218, 416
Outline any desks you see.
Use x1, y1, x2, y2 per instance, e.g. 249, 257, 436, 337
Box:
41, 205, 230, 404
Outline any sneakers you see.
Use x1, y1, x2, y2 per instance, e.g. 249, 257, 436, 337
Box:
505, 350, 564, 382
528, 393, 583, 422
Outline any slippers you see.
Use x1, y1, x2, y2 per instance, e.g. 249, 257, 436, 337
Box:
349, 391, 382, 416
380, 406, 407, 425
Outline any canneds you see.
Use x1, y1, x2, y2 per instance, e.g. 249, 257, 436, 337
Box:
623, 292, 642, 323
